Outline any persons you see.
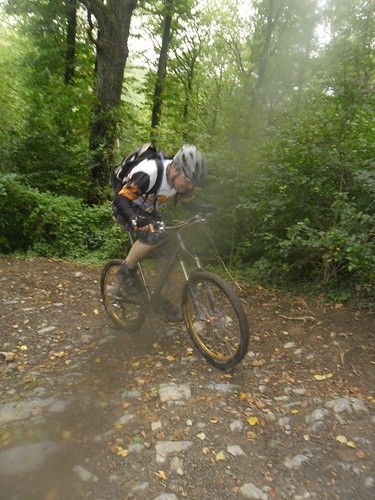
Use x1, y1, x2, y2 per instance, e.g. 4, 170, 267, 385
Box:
111, 143, 218, 321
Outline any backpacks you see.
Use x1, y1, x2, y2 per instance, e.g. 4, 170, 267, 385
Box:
108, 141, 181, 211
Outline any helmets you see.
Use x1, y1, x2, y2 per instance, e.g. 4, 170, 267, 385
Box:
173, 144, 208, 187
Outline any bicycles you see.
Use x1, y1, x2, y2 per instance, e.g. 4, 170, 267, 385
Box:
99, 211, 250, 369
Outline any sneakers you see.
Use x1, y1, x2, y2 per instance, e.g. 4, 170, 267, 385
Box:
152, 298, 183, 322
114, 264, 143, 297
